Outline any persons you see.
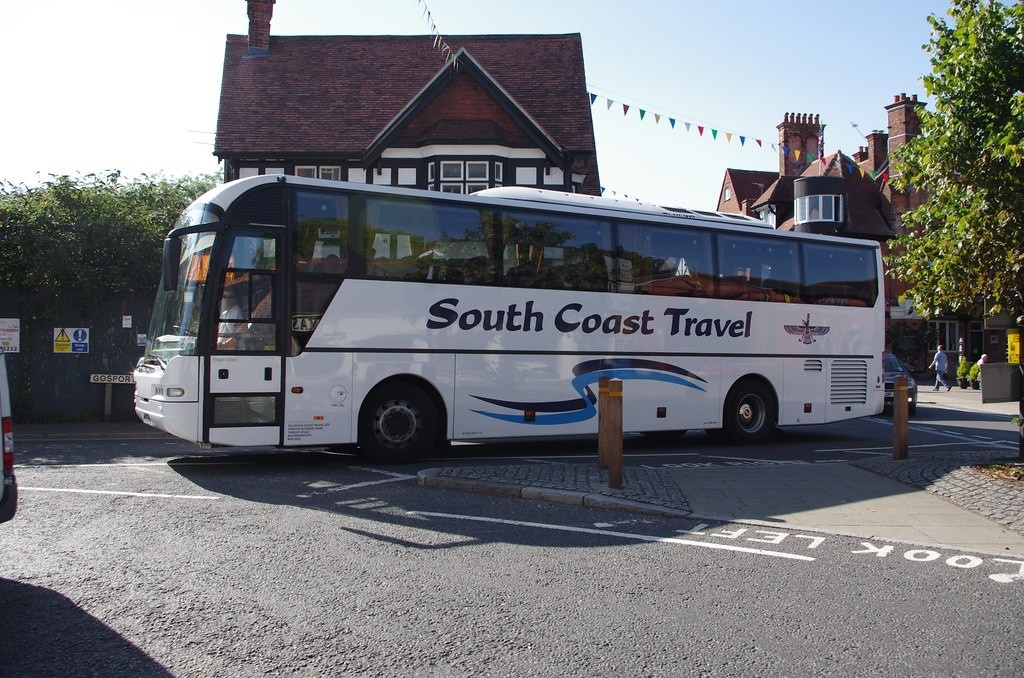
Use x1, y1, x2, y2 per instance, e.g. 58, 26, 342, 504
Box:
367, 245, 582, 291
977, 354, 988, 371
216, 285, 248, 350
928, 345, 952, 392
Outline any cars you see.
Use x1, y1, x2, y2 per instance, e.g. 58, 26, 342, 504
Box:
882, 353, 918, 415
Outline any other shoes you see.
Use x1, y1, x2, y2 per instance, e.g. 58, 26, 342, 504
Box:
933, 388, 939, 391
946, 386, 953, 392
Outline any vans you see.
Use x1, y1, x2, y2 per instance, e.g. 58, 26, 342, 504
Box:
0, 344, 18, 522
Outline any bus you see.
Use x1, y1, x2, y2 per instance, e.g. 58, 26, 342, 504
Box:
132, 174, 887, 468
